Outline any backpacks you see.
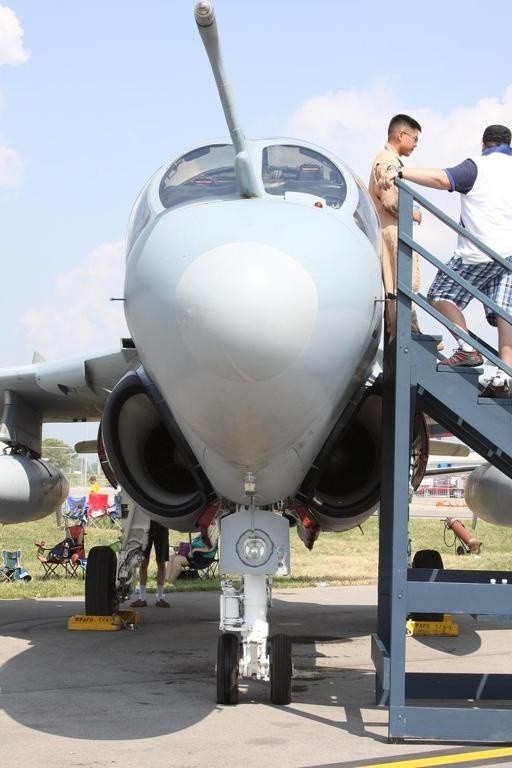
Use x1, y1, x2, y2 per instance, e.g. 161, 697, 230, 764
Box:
46, 537, 75, 563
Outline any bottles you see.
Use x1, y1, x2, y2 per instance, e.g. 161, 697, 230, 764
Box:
63, 542, 70, 558
37, 542, 46, 557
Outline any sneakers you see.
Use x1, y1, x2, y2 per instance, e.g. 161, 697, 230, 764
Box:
479, 379, 511, 399
130, 598, 147, 607
439, 349, 484, 366
155, 598, 170, 608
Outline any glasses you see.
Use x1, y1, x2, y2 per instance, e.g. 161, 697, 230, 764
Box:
404, 131, 418, 143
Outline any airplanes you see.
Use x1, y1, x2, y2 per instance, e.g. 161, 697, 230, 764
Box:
2, 2, 510, 705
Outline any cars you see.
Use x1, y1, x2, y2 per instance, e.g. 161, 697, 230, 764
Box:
413, 476, 465, 497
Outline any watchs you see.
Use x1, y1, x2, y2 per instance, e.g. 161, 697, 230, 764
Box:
395, 165, 403, 179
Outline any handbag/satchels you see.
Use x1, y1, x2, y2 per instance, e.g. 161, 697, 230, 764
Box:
174, 542, 189, 556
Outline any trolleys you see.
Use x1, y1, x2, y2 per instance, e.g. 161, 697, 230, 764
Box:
438, 518, 482, 555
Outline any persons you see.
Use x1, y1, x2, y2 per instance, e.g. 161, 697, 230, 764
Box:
89, 476, 100, 494
369, 114, 444, 351
131, 520, 171, 607
377, 124, 512, 398
165, 535, 218, 582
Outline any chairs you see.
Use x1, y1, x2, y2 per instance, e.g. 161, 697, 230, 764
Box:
181, 538, 219, 581
61, 493, 129, 532
34, 541, 89, 580
0, 550, 22, 586
